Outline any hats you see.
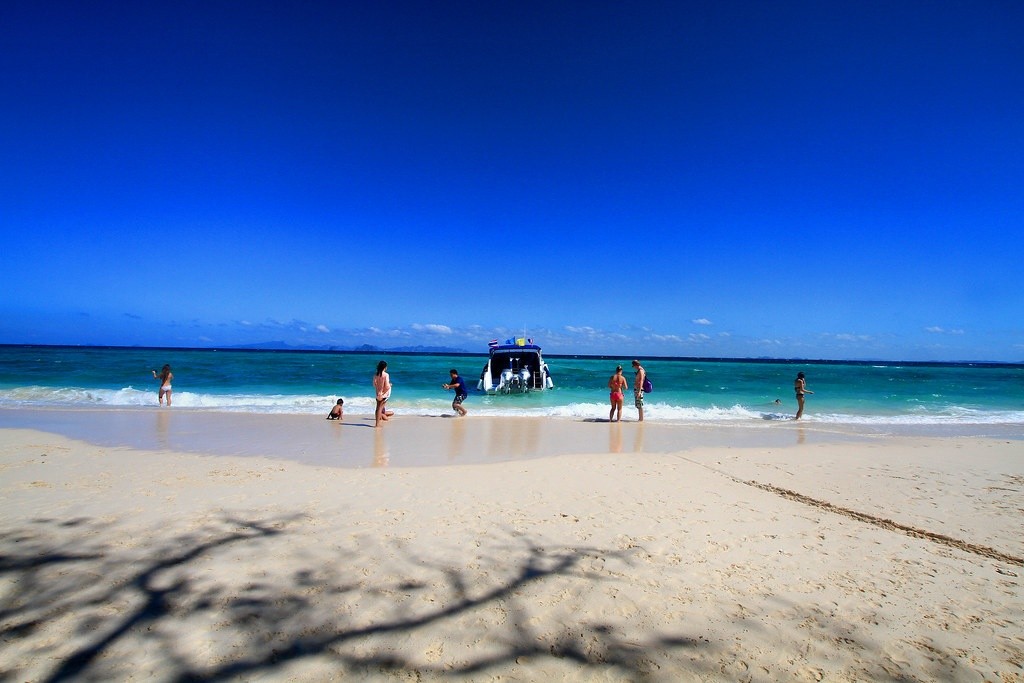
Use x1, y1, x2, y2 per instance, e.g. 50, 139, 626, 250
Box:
632, 360, 640, 367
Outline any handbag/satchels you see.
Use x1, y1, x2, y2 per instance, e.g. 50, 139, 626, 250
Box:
643, 376, 652, 393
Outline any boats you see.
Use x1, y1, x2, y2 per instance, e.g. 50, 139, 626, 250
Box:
484, 343, 547, 395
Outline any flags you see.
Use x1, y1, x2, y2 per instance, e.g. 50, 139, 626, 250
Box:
488, 336, 533, 350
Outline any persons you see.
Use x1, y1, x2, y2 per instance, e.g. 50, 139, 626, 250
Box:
373, 361, 394, 428
608, 365, 628, 422
631, 360, 647, 421
152, 364, 173, 407
326, 398, 344, 420
793, 371, 813, 420
443, 369, 469, 417
